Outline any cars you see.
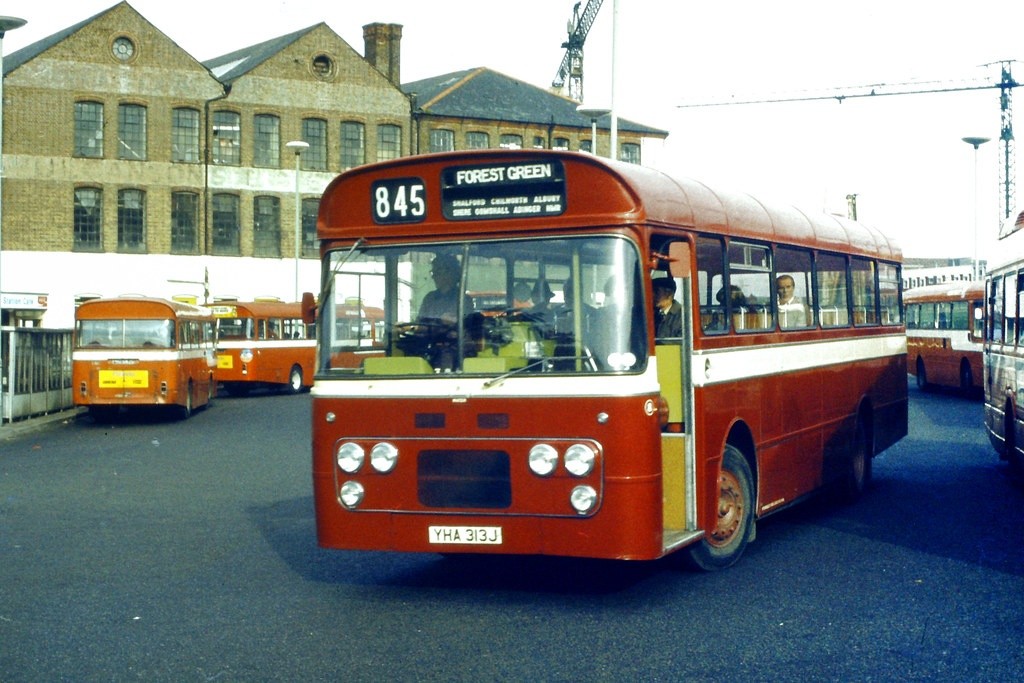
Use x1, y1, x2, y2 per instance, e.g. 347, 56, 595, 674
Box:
896, 280, 984, 395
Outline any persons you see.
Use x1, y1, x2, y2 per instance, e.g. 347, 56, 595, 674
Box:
763, 275, 813, 328
649, 276, 684, 344
395, 253, 475, 335
248, 318, 304, 340
523, 273, 615, 336
700, 285, 761, 332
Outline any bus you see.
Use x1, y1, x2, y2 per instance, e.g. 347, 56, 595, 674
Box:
311, 148, 910, 575
199, 296, 388, 394
457, 284, 616, 371
71, 296, 219, 416
983, 209, 1024, 474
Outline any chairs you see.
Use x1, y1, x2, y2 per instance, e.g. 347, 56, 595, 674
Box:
360, 291, 878, 379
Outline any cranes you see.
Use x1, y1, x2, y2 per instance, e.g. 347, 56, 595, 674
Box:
542, 0, 607, 106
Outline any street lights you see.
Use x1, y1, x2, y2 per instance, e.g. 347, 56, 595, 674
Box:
0, 15, 29, 309
962, 134, 992, 280
285, 139, 310, 337
